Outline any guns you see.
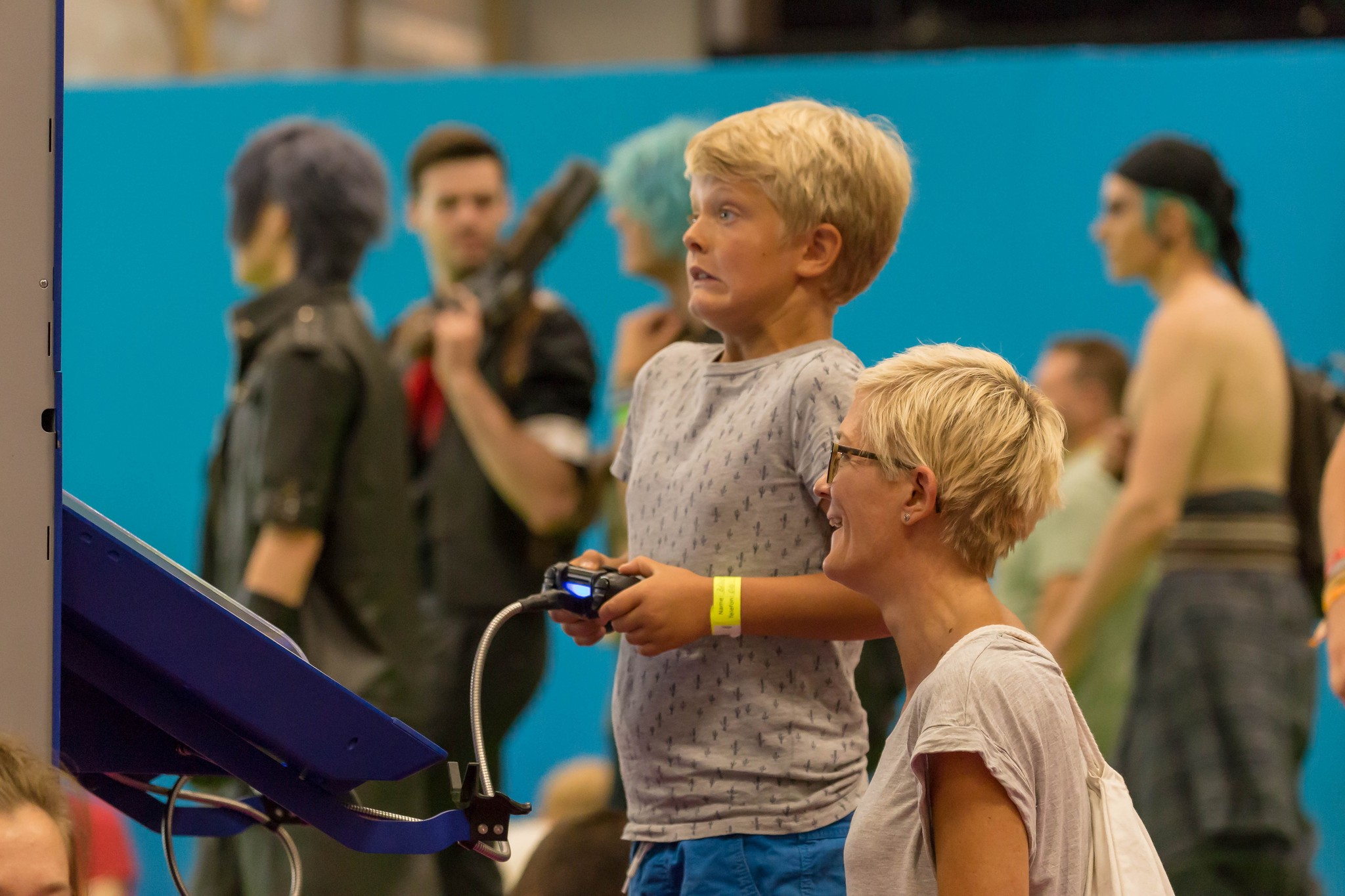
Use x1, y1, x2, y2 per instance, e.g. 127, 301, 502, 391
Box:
405, 158, 605, 374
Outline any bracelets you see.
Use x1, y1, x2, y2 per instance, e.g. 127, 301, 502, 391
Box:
710, 572, 745, 642
1320, 544, 1345, 614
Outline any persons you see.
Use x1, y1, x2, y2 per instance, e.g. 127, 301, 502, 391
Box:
370, 124, 602, 793
181, 123, 510, 896
510, 97, 911, 896
1315, 379, 1345, 702
809, 345, 1106, 896
985, 331, 1162, 775
1047, 134, 1318, 896
0, 729, 141, 896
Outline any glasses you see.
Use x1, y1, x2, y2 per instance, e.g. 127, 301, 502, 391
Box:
824, 441, 940, 516
